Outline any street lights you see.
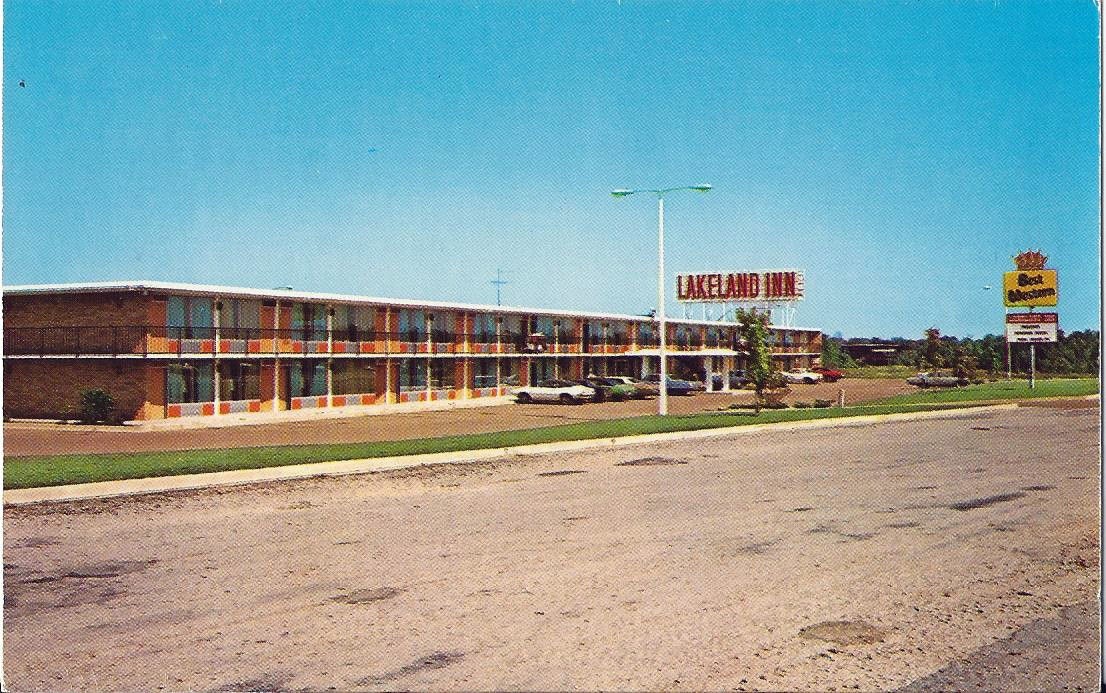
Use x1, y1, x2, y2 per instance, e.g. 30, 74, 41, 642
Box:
982, 285, 1012, 381
612, 184, 712, 415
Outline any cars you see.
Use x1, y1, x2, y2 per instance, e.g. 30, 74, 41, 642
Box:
711, 366, 846, 391
504, 367, 705, 404
905, 370, 971, 389
